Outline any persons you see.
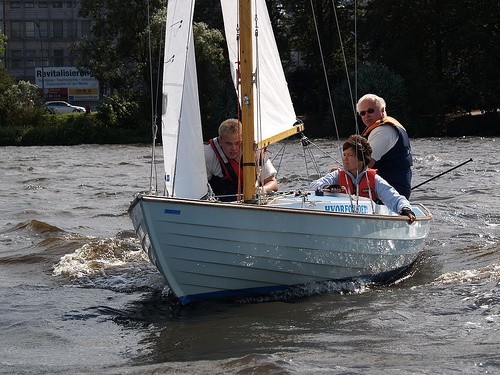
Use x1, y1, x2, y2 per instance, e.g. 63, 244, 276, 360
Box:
356, 94, 413, 205
309, 135, 416, 225
204, 119, 279, 202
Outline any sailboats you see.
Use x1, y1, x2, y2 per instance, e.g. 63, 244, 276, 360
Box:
126, 0, 435, 305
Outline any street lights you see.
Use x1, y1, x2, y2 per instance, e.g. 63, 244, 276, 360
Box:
34, 17, 45, 99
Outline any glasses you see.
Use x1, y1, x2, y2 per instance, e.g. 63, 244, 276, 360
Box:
358, 108, 374, 117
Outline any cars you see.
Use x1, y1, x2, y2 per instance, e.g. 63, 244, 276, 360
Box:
38, 101, 86, 116
